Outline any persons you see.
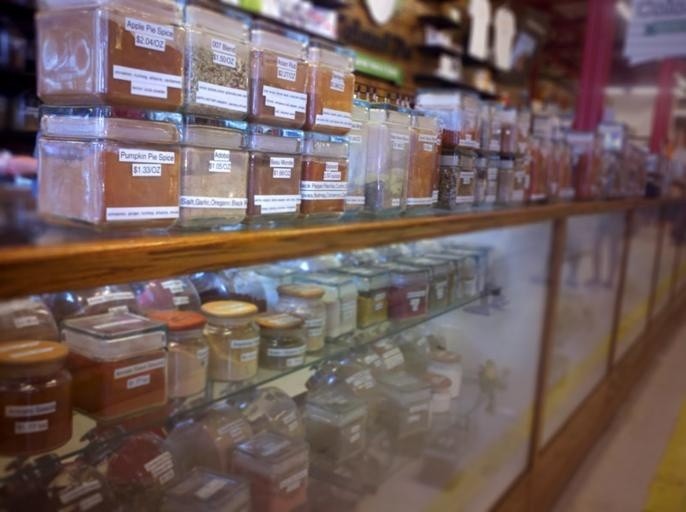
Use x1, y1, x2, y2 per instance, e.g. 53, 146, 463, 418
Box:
666, 115, 686, 247
0, 146, 39, 176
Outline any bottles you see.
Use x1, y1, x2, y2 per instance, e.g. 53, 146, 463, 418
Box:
33, 0, 668, 233
0, 235, 508, 511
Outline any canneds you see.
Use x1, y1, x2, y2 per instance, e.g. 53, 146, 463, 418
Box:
201, 298, 260, 382
146, 308, 209, 399
256, 312, 307, 372
0, 339, 73, 458
274, 283, 326, 354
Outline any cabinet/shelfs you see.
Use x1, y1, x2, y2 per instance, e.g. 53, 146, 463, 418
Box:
1, 188, 685, 512
